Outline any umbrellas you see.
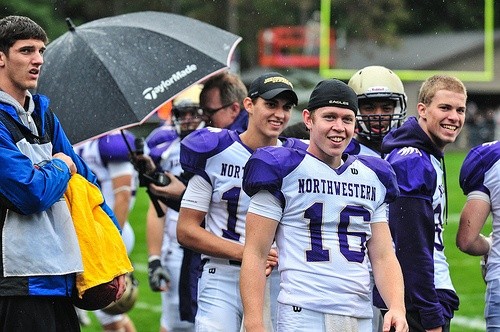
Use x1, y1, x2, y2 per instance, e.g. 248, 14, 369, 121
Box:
28, 11, 242, 218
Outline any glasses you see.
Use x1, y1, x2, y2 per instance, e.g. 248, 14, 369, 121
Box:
201, 100, 237, 117
170, 107, 204, 119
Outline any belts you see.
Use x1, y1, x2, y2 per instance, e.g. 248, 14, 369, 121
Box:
203, 257, 242, 266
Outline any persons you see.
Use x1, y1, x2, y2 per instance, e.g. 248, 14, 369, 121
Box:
175, 70, 299, 332
347, 65, 408, 146
188, 68, 250, 132
378, 73, 468, 332
0, 15, 135, 332
63, 129, 140, 332
145, 83, 208, 332
240, 77, 411, 332
455, 140, 500, 332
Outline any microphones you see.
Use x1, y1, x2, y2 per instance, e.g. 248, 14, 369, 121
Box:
134, 138, 145, 174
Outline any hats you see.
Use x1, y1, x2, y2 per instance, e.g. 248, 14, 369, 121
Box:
308, 78, 358, 118
247, 72, 299, 107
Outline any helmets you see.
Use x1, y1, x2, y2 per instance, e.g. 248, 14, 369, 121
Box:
171, 83, 205, 108
347, 65, 407, 142
